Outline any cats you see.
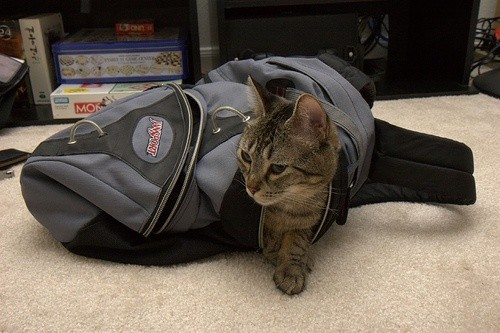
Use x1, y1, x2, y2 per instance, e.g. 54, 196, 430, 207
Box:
234, 73, 343, 296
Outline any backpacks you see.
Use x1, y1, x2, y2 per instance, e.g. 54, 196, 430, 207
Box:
19, 52, 477, 266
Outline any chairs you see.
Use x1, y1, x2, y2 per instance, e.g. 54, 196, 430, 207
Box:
0, 48, 32, 181
